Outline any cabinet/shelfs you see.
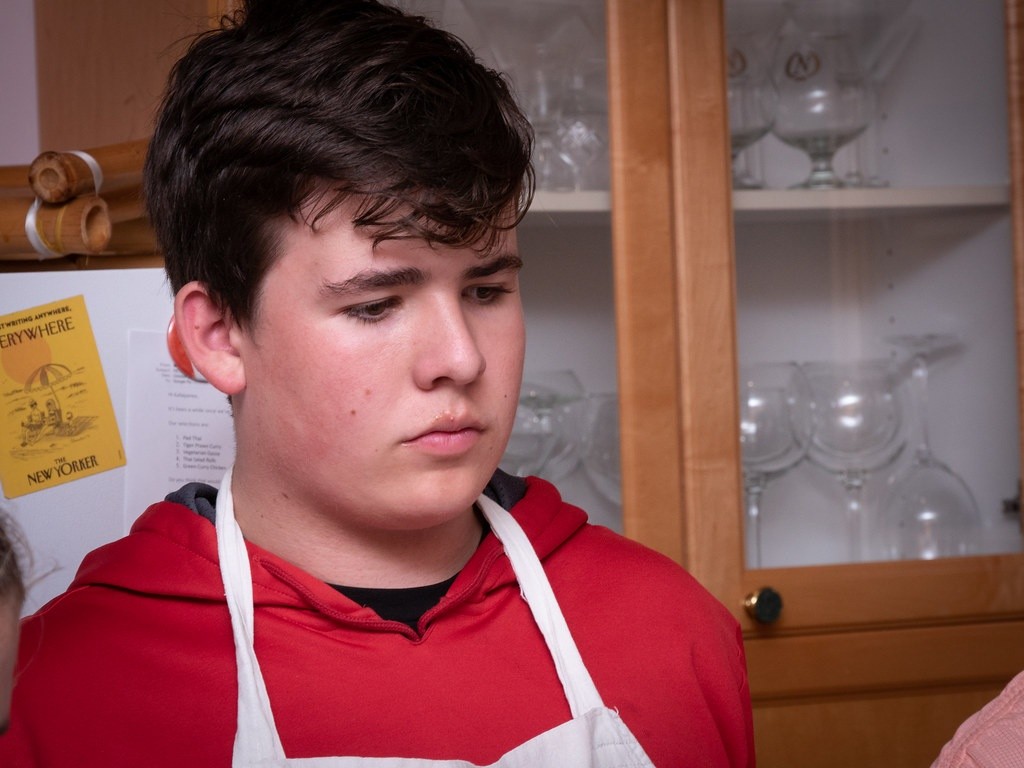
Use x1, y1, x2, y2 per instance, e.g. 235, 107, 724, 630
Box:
210, 1, 1024, 767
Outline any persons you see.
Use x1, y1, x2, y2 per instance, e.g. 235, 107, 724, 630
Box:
0, 0, 759, 768
0, 505, 36, 730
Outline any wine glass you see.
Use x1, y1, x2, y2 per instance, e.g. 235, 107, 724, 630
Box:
460, 1, 918, 188
495, 333, 980, 564
